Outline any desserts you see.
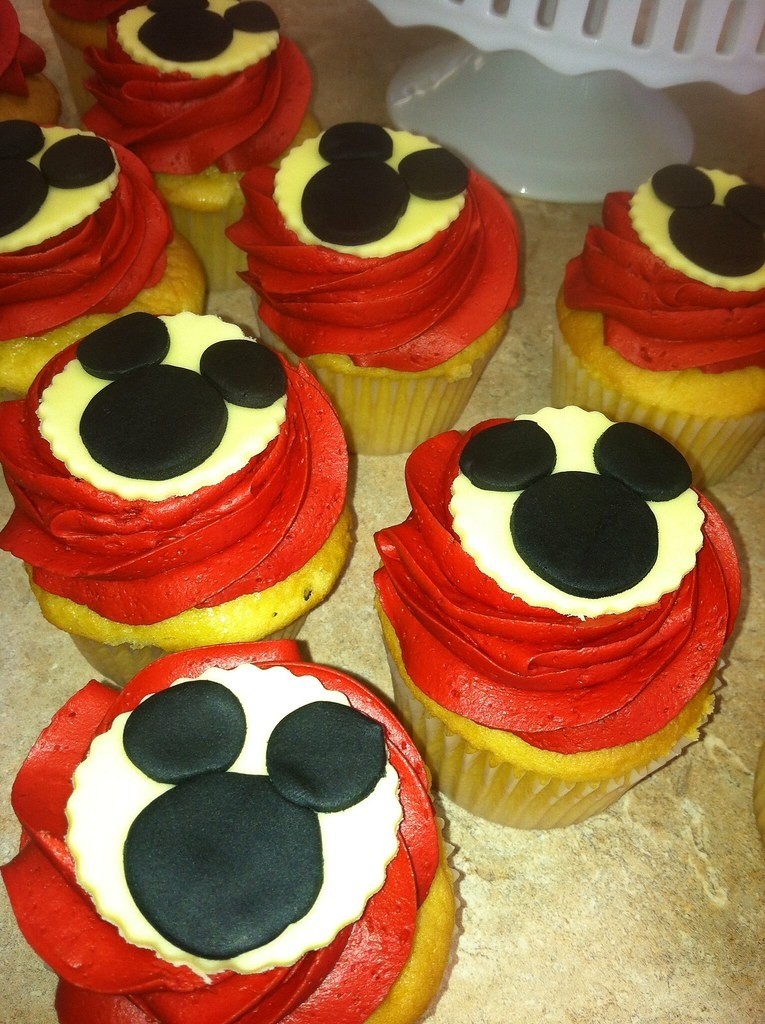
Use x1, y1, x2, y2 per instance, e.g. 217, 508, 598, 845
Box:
0, 0, 765, 1024
